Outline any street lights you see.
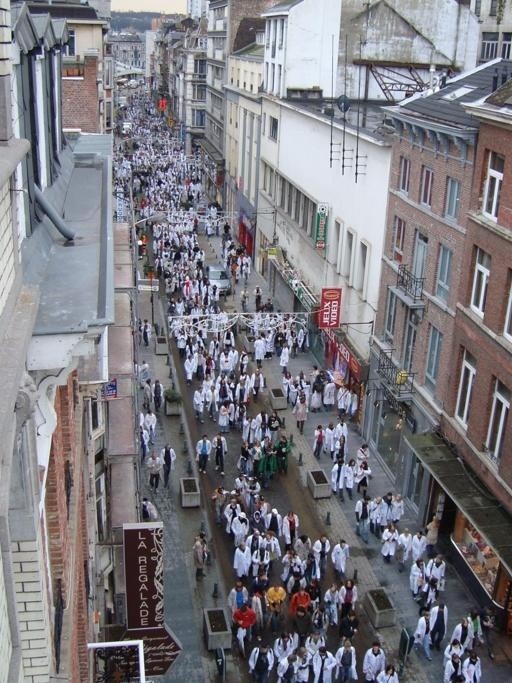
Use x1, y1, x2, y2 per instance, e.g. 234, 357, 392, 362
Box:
147, 266, 155, 325
114, 104, 126, 121
134, 213, 165, 342
116, 136, 145, 184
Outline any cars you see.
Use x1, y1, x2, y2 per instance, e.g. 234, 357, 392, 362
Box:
118, 96, 127, 106
118, 77, 145, 89
206, 264, 231, 295
121, 121, 132, 135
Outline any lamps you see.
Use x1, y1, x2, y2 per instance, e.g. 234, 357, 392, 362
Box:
333, 318, 375, 344
365, 381, 408, 422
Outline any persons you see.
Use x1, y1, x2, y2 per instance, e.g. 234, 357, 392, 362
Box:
116, 91, 498, 682
